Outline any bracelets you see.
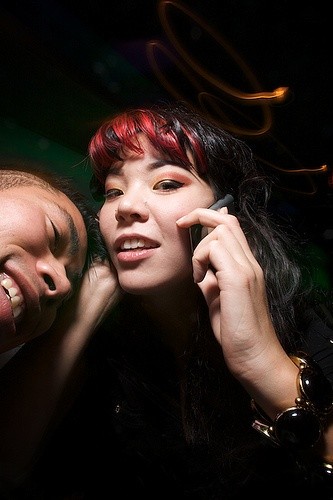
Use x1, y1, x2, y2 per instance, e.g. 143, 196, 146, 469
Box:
248, 348, 333, 471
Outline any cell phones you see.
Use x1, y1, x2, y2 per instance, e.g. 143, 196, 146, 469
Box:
189, 194, 236, 256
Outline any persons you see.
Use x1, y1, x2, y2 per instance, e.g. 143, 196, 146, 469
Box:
0, 164, 108, 355
0, 103, 333, 500
